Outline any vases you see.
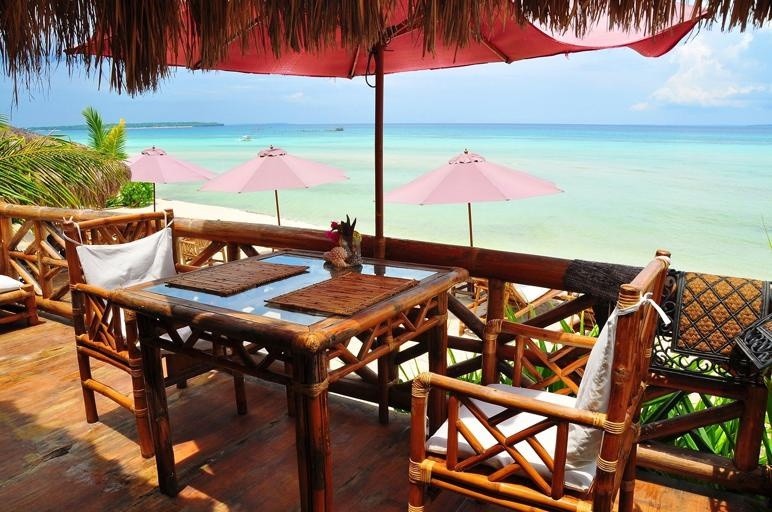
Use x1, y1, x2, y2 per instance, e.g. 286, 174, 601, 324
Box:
339, 234, 362, 265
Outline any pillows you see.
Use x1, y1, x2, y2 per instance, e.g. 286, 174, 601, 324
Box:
75, 230, 181, 339
0, 274, 24, 293
555, 302, 618, 467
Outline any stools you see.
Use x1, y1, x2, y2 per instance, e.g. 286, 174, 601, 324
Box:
1, 275, 38, 329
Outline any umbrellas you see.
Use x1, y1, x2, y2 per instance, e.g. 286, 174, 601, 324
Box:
384, 147, 567, 248
59, 0, 716, 261
196, 144, 350, 225
125, 144, 215, 214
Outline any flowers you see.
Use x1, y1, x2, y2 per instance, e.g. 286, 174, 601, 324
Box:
323, 214, 356, 253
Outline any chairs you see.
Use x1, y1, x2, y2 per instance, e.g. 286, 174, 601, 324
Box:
64, 209, 247, 459
408, 251, 671, 512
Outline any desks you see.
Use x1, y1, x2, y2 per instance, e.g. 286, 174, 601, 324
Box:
109, 248, 468, 512
